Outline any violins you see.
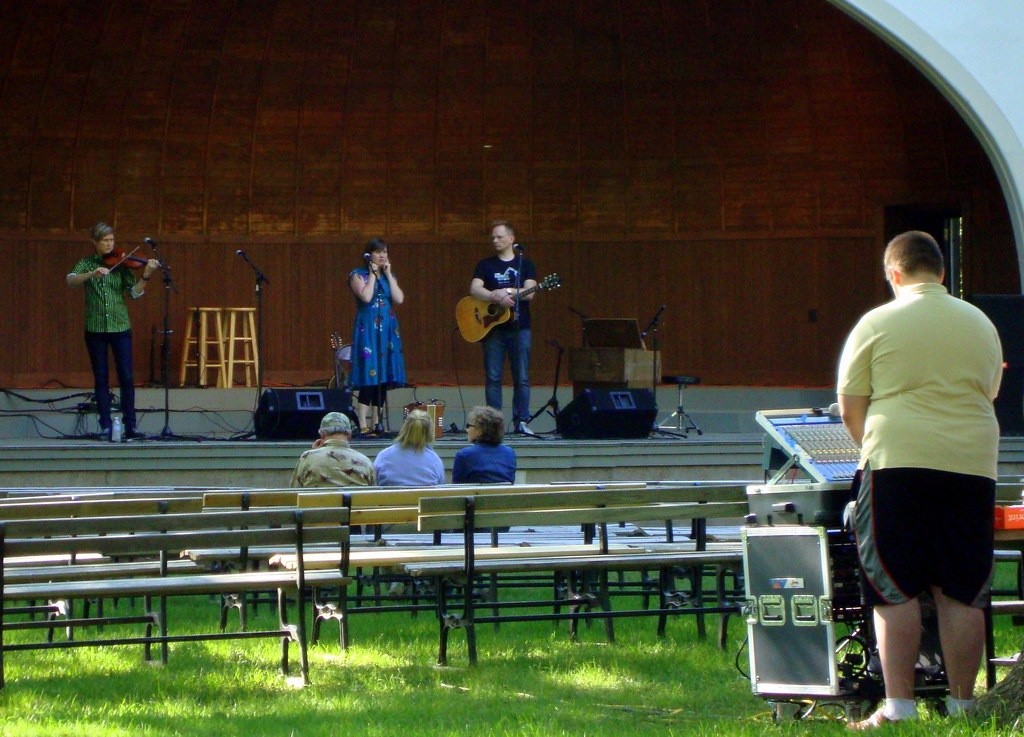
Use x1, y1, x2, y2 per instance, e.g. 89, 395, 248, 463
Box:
104, 247, 176, 274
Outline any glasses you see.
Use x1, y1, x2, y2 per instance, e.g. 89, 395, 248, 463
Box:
466, 423, 474, 428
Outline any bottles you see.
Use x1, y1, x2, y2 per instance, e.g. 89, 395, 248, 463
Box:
112, 417, 121, 442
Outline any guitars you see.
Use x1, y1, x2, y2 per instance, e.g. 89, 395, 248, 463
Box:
456, 272, 561, 342
327, 330, 343, 390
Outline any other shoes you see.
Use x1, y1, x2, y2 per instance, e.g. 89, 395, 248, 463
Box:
848, 706, 902, 731
516, 422, 534, 435
125, 430, 145, 438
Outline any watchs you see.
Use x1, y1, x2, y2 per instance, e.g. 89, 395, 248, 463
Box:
504, 287, 511, 295
141, 273, 150, 281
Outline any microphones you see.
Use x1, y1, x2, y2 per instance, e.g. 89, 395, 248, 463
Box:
144, 237, 157, 245
235, 249, 246, 257
364, 253, 372, 262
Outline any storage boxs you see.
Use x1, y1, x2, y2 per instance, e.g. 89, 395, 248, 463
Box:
994, 505, 1024, 529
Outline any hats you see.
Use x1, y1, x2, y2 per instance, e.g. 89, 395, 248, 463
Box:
320, 412, 352, 434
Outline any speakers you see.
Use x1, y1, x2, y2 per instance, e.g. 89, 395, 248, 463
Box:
252, 388, 354, 442
556, 387, 659, 439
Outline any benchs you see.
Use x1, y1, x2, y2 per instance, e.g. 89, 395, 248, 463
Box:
0, 475, 1024, 693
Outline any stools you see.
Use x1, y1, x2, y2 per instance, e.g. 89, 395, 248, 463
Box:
217, 308, 259, 389
179, 308, 226, 389
655, 376, 703, 436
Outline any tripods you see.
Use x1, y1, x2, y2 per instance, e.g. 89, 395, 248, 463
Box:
139, 245, 201, 441
526, 339, 566, 425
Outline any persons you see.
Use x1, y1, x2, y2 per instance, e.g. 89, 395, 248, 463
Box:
450, 405, 517, 485
837, 233, 1003, 728
66, 222, 159, 441
288, 411, 376, 535
348, 239, 404, 439
373, 410, 448, 486
470, 219, 538, 436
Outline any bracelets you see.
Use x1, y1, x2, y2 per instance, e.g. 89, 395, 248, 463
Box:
88, 271, 92, 279
